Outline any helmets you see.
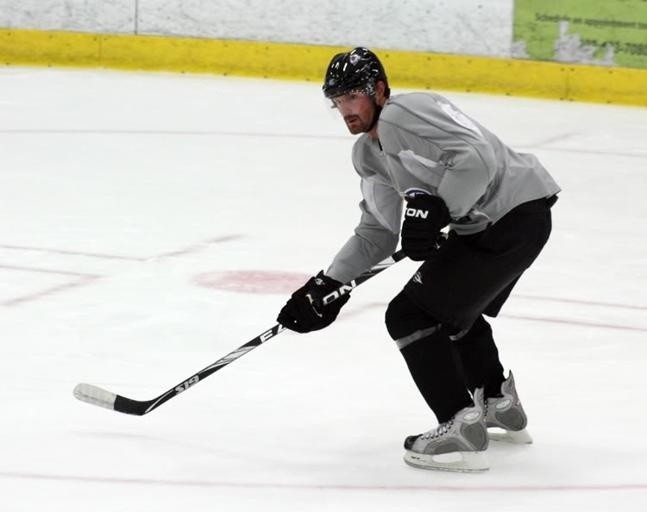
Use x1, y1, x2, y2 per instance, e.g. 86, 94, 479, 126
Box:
322, 46, 389, 103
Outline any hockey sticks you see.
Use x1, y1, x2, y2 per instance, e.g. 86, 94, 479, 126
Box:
72, 229, 450, 416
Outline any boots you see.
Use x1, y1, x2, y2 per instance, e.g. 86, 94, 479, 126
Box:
404, 370, 527, 455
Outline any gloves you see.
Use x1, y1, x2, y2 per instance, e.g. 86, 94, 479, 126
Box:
401, 194, 453, 260
276, 269, 350, 333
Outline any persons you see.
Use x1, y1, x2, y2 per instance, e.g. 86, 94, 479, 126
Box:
277, 47, 561, 456
